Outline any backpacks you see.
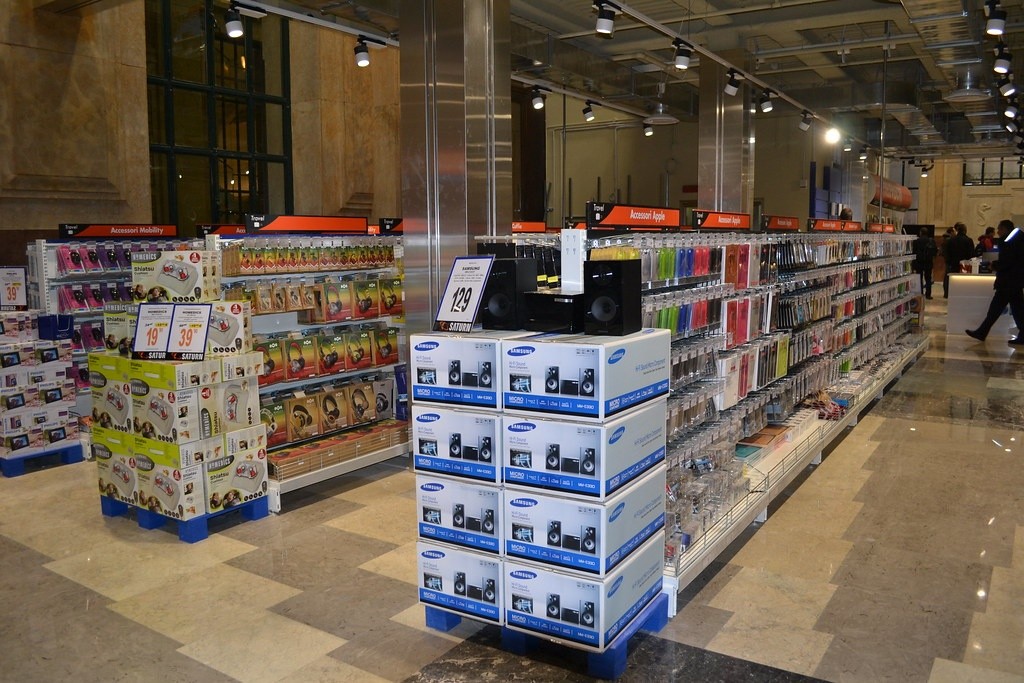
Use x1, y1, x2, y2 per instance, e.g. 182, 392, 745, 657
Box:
975, 241, 985, 257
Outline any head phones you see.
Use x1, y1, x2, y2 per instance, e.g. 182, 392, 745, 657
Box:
257, 281, 397, 436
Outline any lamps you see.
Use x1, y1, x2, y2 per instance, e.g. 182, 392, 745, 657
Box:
224, 2, 267, 37
945, 0, 1023, 148
725, 68, 746, 95
919, 164, 935, 177
758, 87, 777, 113
799, 110, 868, 161
353, 35, 387, 68
591, 0, 623, 34
642, 38, 695, 137
582, 100, 600, 122
529, 84, 554, 110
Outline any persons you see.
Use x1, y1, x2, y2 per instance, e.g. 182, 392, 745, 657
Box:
913, 228, 937, 299
941, 221, 974, 298
980, 227, 995, 251
965, 220, 1024, 344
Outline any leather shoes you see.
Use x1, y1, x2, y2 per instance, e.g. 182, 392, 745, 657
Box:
965, 329, 987, 342
1008, 334, 1023, 345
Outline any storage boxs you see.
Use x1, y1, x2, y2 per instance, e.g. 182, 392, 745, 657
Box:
0, 239, 408, 520
410, 326, 671, 653
782, 409, 818, 441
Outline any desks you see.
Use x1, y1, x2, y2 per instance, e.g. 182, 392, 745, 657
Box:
946, 273, 1010, 338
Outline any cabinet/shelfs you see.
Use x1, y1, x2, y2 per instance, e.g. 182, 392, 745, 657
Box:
208, 233, 413, 516
26, 240, 213, 460
474, 232, 930, 620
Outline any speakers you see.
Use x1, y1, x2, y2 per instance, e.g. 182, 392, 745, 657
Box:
445, 360, 596, 629
584, 259, 642, 336
524, 290, 584, 334
481, 258, 537, 330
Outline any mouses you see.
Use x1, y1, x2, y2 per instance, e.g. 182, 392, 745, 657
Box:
91, 327, 102, 341
72, 249, 134, 303
79, 368, 89, 381
73, 330, 81, 344
